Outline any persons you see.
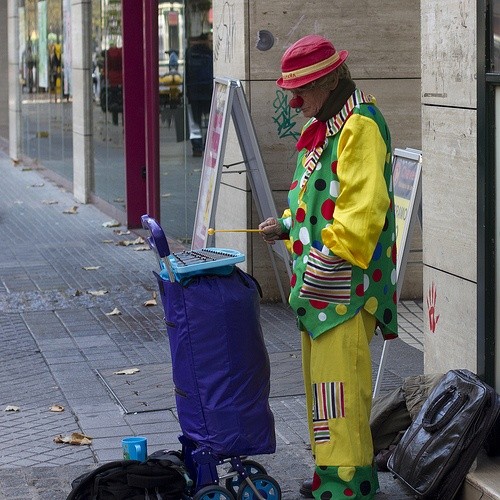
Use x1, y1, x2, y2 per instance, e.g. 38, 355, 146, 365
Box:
19, 35, 220, 158
258, 35, 398, 494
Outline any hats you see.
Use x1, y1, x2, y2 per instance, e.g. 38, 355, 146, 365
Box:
276, 35, 349, 90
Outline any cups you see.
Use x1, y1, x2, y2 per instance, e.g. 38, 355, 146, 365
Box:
121, 437, 147, 463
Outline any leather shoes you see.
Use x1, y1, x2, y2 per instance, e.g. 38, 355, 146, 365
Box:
300, 480, 314, 498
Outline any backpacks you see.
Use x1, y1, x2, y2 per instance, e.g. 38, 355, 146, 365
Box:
66, 448, 192, 500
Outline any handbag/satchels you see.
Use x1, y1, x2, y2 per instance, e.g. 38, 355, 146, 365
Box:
388, 369, 500, 500
